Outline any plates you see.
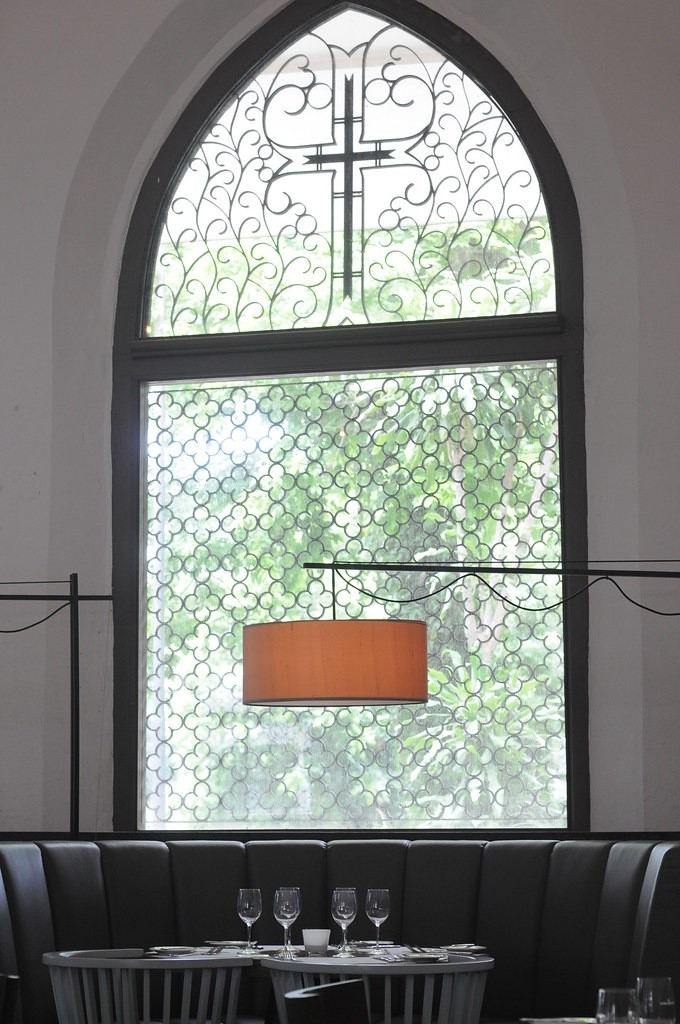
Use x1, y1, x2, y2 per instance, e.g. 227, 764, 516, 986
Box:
440, 946, 487, 952
379, 947, 448, 960
150, 947, 195, 954
349, 941, 394, 945
205, 941, 258, 946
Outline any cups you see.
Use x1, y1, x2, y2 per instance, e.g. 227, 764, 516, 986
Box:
596, 977, 676, 1024
302, 929, 331, 957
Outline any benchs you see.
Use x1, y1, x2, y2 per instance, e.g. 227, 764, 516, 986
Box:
0, 838, 680, 1024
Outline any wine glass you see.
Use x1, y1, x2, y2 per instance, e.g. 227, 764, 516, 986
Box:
272, 887, 301, 958
365, 889, 391, 954
236, 889, 263, 955
331, 887, 358, 958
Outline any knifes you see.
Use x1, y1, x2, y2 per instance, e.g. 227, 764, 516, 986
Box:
404, 944, 419, 953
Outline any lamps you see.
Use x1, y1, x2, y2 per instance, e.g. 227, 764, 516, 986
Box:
244, 568, 429, 708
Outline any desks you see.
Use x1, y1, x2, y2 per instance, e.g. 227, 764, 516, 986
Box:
43, 945, 496, 1024
